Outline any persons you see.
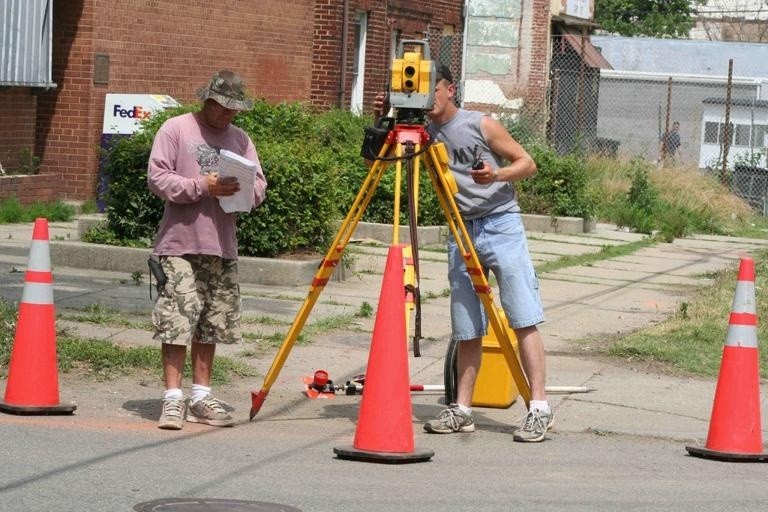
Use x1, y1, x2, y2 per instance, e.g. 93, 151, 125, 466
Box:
371, 57, 558, 444
142, 68, 268, 431
657, 121, 683, 157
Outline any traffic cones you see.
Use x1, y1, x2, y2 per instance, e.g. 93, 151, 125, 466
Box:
330, 244, 434, 462
0, 213, 77, 418
680, 255, 767, 462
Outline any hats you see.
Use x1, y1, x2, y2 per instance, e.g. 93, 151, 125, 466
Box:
435, 62, 452, 83
195, 69, 255, 111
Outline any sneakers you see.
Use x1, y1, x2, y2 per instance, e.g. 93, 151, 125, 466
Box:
424, 402, 476, 434
513, 406, 556, 443
158, 393, 189, 430
185, 393, 237, 428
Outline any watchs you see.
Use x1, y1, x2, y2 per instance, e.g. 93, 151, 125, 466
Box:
490, 169, 500, 184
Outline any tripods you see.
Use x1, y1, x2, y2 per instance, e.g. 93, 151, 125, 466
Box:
249, 124, 531, 423
